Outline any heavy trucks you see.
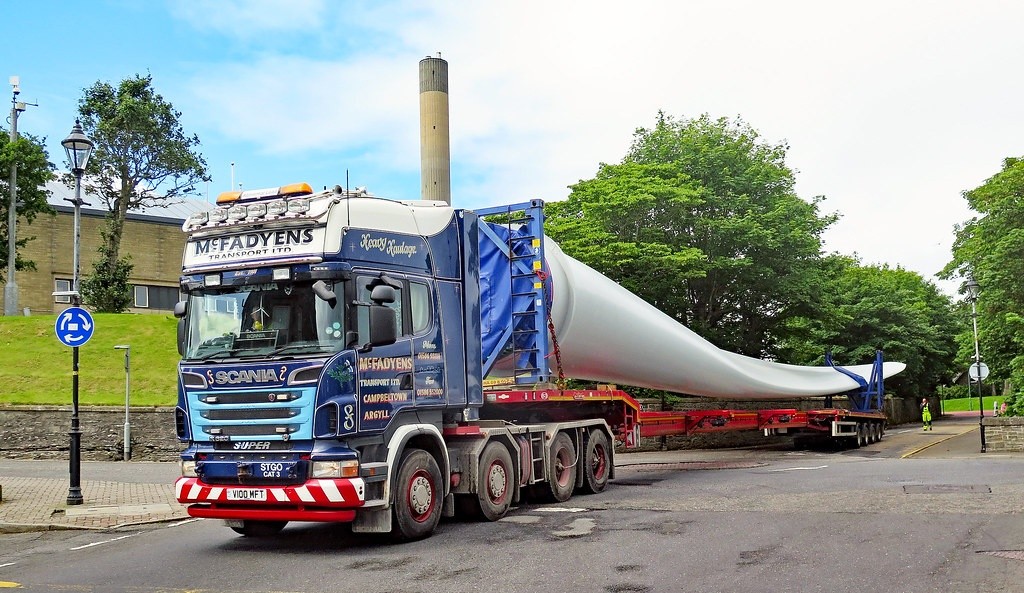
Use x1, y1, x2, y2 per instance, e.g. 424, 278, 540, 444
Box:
173, 168, 889, 541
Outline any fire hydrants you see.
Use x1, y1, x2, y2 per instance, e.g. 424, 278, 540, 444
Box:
993, 401, 998, 415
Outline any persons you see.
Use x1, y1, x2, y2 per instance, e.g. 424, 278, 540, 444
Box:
1001, 402, 1006, 413
245, 306, 270, 339
920, 398, 934, 431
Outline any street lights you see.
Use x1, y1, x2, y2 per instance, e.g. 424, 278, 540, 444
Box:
60, 118, 96, 506
967, 276, 986, 453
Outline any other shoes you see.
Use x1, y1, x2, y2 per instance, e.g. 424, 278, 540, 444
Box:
923, 426, 927, 431
930, 426, 932, 430
